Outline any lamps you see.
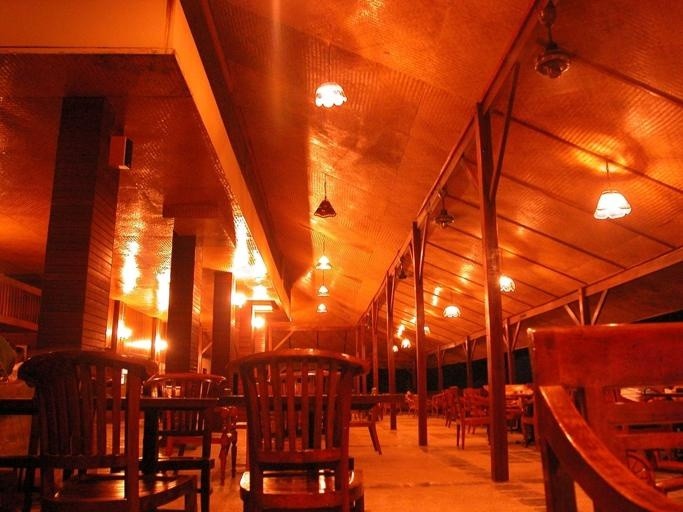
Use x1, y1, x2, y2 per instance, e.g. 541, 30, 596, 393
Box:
532, 3, 571, 79
435, 186, 454, 230
314, 240, 333, 314
314, 42, 347, 108
594, 158, 633, 221
314, 173, 336, 218
442, 290, 461, 319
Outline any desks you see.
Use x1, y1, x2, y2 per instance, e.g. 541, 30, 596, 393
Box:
0, 391, 411, 511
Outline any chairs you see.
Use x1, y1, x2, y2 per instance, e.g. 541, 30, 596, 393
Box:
521, 325, 682, 511
12, 348, 200, 512
140, 371, 226, 501
227, 347, 369, 511
397, 383, 535, 452
